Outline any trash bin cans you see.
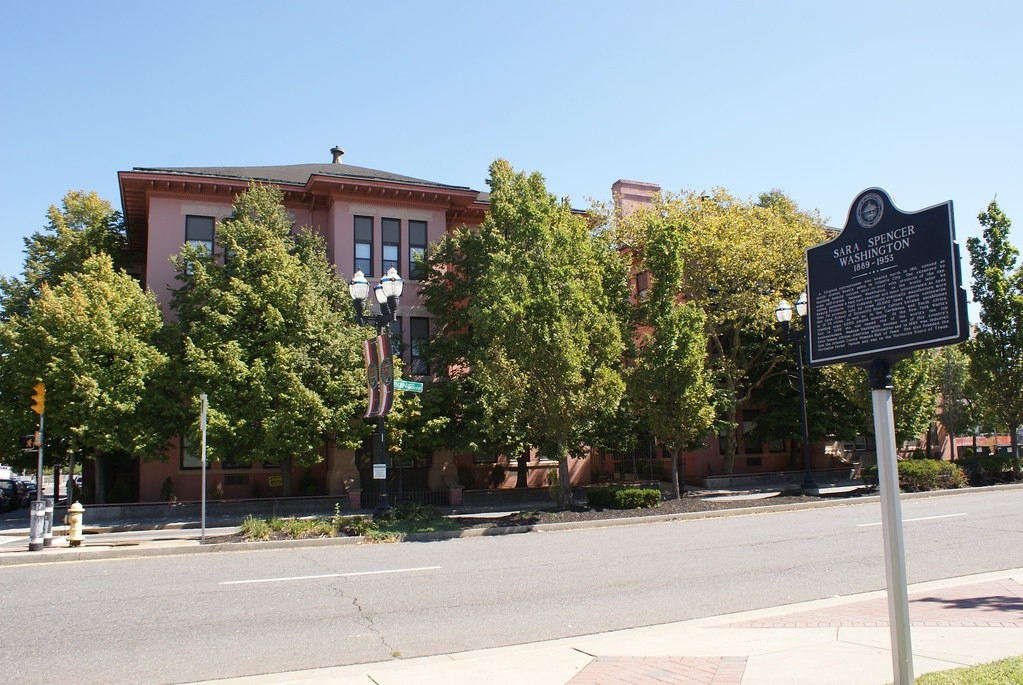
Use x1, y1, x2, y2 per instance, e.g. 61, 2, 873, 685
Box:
30, 497, 54, 549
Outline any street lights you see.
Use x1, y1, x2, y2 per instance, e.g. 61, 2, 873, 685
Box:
350, 263, 407, 507
775, 292, 818, 488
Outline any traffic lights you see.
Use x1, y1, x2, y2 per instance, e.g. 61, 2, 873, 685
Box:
31, 382, 45, 414
19, 435, 34, 450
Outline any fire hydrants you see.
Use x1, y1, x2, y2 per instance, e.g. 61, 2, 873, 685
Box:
62, 501, 85, 546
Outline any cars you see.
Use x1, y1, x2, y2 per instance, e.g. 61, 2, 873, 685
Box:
0, 476, 45, 514
75, 476, 82, 495
